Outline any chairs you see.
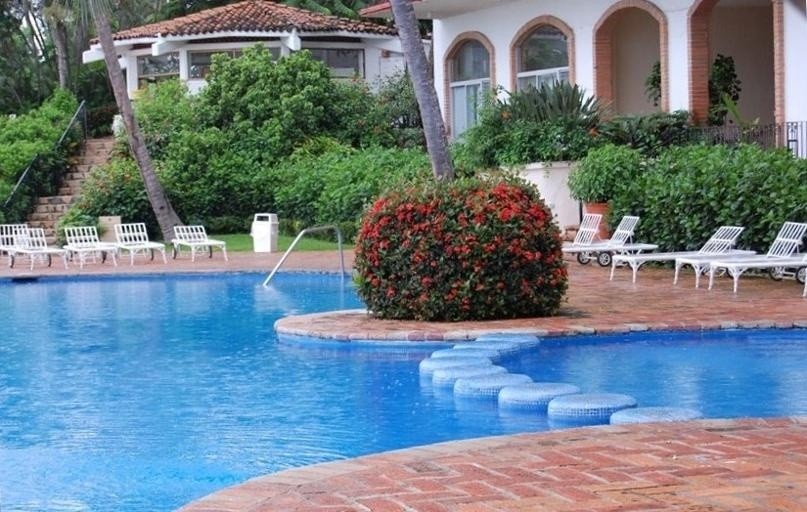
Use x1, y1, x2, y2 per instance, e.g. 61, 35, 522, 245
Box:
560, 213, 807, 298
0, 221, 229, 271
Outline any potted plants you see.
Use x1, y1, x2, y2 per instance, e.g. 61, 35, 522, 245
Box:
566, 143, 640, 243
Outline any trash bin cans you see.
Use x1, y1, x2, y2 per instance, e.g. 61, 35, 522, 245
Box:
252, 213, 279, 253
111, 115, 122, 138
